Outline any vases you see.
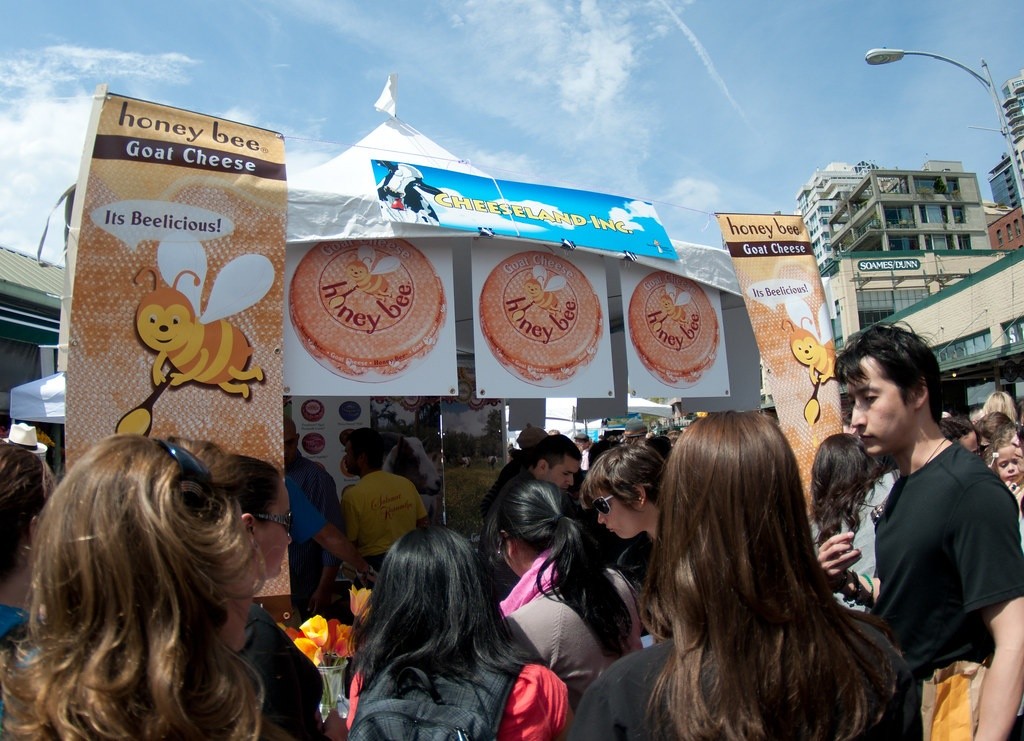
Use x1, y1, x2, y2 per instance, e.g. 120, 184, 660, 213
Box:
318, 656, 349, 723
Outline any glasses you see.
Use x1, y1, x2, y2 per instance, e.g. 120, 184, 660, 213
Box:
1015, 421, 1024, 438
156, 436, 211, 516
592, 490, 621, 515
246, 511, 292, 534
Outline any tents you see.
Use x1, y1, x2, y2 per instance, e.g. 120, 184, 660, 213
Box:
11, 117, 761, 503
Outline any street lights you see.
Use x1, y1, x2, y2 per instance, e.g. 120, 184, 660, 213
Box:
864, 45, 1024, 218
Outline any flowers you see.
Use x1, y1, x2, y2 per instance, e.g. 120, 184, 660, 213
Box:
277, 583, 374, 717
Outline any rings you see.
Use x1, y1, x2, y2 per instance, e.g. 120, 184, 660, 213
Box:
1012, 484, 1016, 489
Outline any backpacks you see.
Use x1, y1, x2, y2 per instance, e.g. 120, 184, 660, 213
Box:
347, 648, 522, 741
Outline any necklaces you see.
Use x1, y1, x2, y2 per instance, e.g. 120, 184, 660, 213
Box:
926, 437, 948, 463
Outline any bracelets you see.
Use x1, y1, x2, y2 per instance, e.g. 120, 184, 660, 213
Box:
355, 565, 374, 577
862, 573, 873, 587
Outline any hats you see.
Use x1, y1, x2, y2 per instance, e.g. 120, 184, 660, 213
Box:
2, 422, 48, 455
574, 433, 588, 444
516, 424, 547, 449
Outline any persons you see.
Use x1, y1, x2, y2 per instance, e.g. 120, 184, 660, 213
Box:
217, 456, 331, 741
807, 432, 899, 613
816, 321, 1023, 741
937, 389, 1024, 550
483, 478, 643, 714
0, 432, 304, 741
346, 525, 574, 740
567, 411, 923, 741
0, 422, 57, 734
282, 412, 443, 694
506, 416, 681, 561
579, 443, 676, 645
478, 436, 583, 600
